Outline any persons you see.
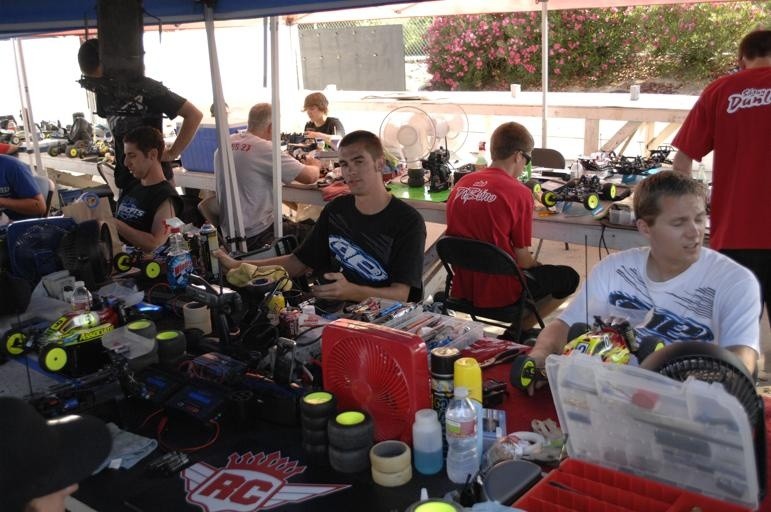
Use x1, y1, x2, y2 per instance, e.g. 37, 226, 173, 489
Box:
77, 36, 205, 164
113, 125, 184, 258
442, 121, 580, 340
670, 28, 771, 323
300, 91, 347, 151
211, 102, 319, 254
0, 152, 47, 223
522, 170, 761, 397
213, 129, 427, 302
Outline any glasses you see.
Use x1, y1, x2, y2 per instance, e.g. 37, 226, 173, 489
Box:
517, 147, 532, 163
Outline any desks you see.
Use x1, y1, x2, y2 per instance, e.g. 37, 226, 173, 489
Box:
15, 152, 682, 281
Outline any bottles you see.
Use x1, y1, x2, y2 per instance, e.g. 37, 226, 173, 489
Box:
71, 282, 92, 314
475, 143, 486, 169
412, 410, 443, 476
168, 225, 192, 286
446, 388, 479, 482
199, 218, 220, 281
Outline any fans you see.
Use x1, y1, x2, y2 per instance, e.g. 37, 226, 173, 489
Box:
640, 343, 761, 425
380, 107, 437, 169
419, 104, 468, 156
320, 315, 433, 449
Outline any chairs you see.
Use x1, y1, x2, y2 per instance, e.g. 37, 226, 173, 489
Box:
408, 279, 423, 304
198, 195, 234, 246
435, 237, 555, 344
97, 161, 123, 202
33, 175, 56, 219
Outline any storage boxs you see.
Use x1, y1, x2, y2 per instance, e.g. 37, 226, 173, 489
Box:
175, 121, 249, 174
509, 351, 761, 511
